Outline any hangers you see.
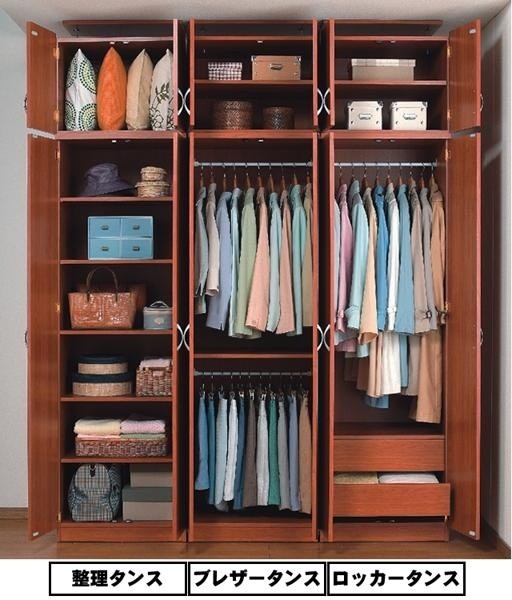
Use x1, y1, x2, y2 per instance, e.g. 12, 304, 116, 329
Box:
197, 162, 310, 193
198, 369, 312, 401
335, 160, 436, 191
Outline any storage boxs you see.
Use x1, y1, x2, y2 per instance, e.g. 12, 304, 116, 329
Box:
348, 59, 416, 79
122, 483, 172, 520
144, 302, 172, 329
252, 56, 301, 80
129, 463, 172, 487
344, 100, 382, 131
389, 101, 427, 130
75, 436, 167, 457
208, 62, 242, 80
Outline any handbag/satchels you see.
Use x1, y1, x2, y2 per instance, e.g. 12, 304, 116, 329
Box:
67, 267, 146, 331
68, 466, 125, 523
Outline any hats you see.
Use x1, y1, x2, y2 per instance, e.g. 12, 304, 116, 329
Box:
81, 163, 134, 196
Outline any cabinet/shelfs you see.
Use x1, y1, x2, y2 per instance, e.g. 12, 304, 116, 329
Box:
24, 19, 177, 542
323, 15, 482, 544
88, 216, 154, 259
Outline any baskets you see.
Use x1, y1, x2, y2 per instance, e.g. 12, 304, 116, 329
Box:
142, 166, 166, 182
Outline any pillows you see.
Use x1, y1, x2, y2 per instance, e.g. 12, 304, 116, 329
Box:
334, 471, 378, 484
63, 49, 96, 130
152, 48, 172, 131
379, 472, 439, 483
126, 47, 152, 131
95, 46, 127, 131
